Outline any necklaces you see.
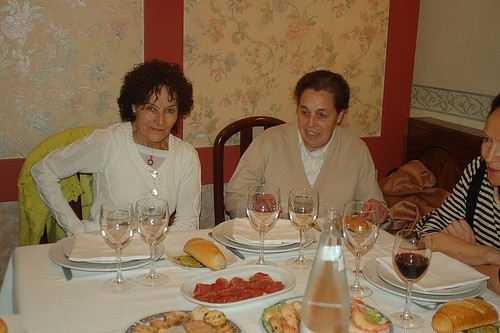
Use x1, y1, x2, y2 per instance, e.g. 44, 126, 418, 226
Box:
134, 121, 168, 144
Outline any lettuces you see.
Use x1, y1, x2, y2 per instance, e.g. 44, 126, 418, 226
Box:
260, 302, 382, 331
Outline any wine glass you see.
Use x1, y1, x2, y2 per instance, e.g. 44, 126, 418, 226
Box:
285, 188, 320, 269
245, 184, 280, 267
134, 199, 170, 285
343, 201, 379, 298
99, 201, 134, 292
391, 229, 434, 329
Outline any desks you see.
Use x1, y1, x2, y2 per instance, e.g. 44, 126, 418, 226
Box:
0, 215, 500, 333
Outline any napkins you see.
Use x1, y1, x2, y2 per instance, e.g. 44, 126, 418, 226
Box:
235, 216, 307, 247
376, 251, 490, 295
68, 231, 170, 264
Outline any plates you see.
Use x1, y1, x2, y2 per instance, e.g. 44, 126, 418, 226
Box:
50, 236, 166, 271
363, 254, 487, 302
211, 218, 316, 253
260, 295, 393, 333
181, 265, 296, 306
126, 310, 242, 333
167, 242, 238, 269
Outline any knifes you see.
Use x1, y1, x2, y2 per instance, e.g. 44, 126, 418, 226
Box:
61, 265, 72, 280
207, 232, 245, 260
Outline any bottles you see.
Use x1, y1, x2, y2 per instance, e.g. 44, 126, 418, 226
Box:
300, 208, 349, 333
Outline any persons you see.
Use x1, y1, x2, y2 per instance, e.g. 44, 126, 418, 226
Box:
30, 58, 201, 236
224, 69, 391, 230
415, 93, 500, 297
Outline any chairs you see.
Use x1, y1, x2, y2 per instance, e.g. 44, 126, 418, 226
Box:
213, 116, 286, 229
18, 124, 106, 248
386, 145, 464, 240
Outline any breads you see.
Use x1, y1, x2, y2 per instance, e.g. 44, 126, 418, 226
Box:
430, 298, 499, 333
134, 305, 235, 333
331, 215, 369, 234
183, 237, 227, 271
